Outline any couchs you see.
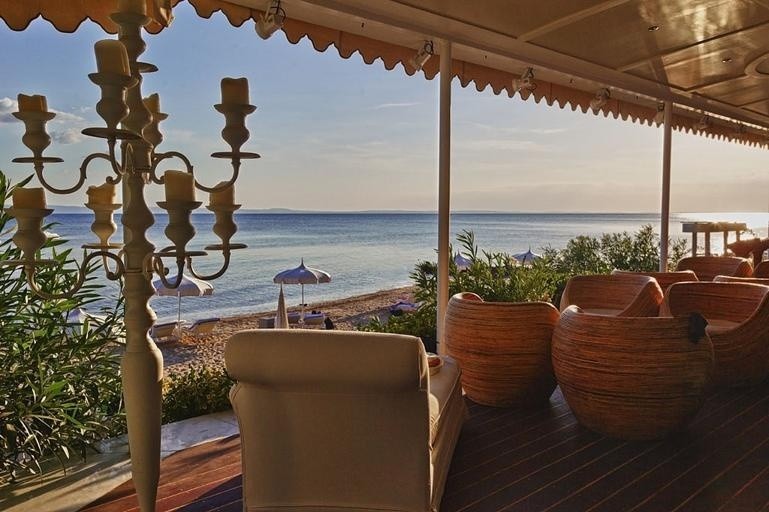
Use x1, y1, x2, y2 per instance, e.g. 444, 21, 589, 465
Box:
228, 329, 464, 512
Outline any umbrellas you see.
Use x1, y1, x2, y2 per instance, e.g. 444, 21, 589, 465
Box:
146, 271, 214, 336
272, 280, 289, 329
272, 256, 332, 326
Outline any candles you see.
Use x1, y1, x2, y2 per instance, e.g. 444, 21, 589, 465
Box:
95, 41, 131, 75
220, 77, 250, 104
18, 94, 48, 112
143, 93, 161, 113
209, 181, 235, 204
164, 171, 197, 201
117, 0, 147, 13
12, 188, 47, 211
88, 182, 116, 205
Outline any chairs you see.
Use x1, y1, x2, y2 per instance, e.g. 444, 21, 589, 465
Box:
558, 274, 664, 318
675, 256, 751, 281
181, 318, 219, 340
443, 292, 560, 407
659, 281, 769, 385
551, 304, 715, 442
286, 313, 299, 322
610, 269, 698, 293
304, 314, 323, 325
714, 260, 769, 287
149, 322, 176, 340
